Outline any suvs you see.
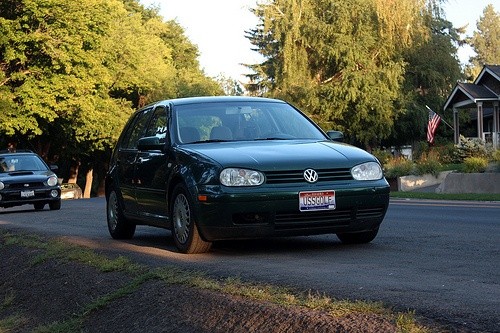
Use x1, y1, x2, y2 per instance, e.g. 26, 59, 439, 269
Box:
104, 96, 389, 254
0, 148, 62, 210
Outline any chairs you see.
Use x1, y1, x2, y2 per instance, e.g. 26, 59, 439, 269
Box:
177, 121, 260, 145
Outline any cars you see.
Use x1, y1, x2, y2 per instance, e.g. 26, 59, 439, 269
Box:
58, 183, 82, 201
